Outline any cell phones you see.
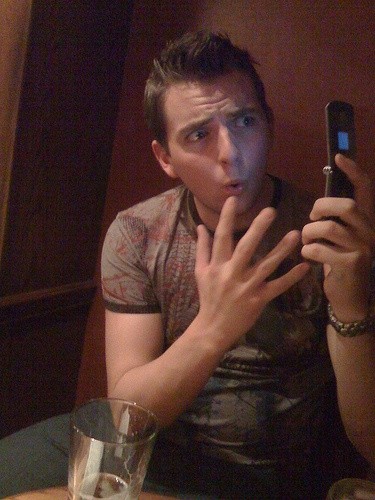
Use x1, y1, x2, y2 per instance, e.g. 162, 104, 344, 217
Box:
304, 100, 357, 265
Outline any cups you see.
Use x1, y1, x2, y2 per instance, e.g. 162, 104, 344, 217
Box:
327, 478, 375, 500
67, 398, 159, 500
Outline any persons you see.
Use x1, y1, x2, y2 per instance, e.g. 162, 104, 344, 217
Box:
0, 30, 375, 500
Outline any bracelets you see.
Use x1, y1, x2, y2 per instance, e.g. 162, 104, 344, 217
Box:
326, 303, 374, 337
